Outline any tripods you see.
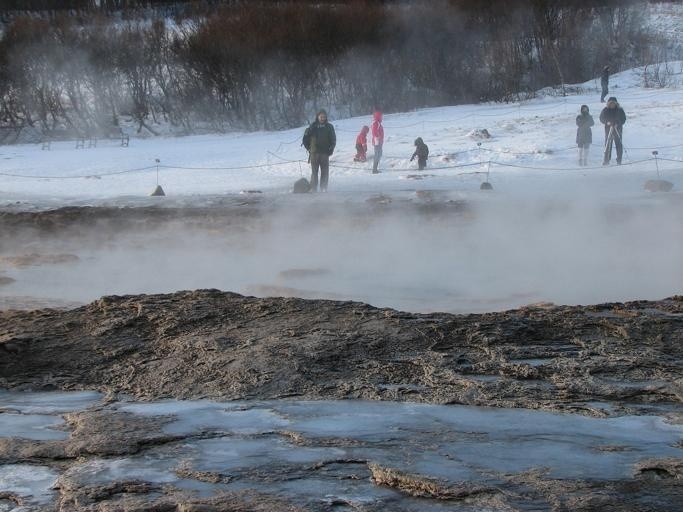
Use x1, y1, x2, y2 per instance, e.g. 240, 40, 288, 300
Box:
603, 126, 632, 166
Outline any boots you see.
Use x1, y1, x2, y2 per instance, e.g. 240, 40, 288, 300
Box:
577, 157, 587, 165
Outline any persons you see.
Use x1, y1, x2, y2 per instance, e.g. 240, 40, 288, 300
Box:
598, 96, 626, 168
369, 110, 387, 175
575, 104, 594, 166
409, 136, 429, 168
353, 125, 369, 162
301, 106, 337, 191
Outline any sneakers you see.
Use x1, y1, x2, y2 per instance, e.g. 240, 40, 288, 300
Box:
307, 186, 329, 193
615, 157, 623, 164
603, 161, 610, 165
